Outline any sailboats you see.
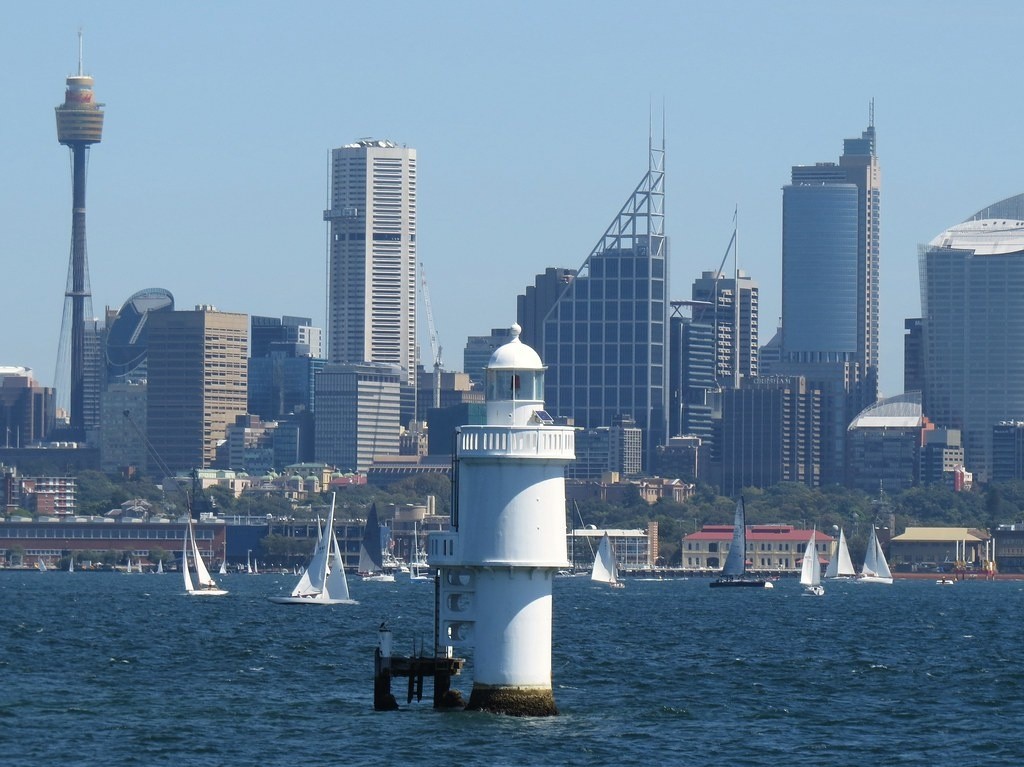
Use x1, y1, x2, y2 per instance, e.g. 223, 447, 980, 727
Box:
247, 558, 262, 576
121, 556, 168, 575
266, 491, 361, 606
407, 522, 427, 580
182, 511, 228, 596
67, 557, 76, 575
856, 523, 894, 584
590, 530, 625, 589
709, 495, 775, 589
357, 502, 395, 582
823, 526, 856, 582
799, 525, 824, 597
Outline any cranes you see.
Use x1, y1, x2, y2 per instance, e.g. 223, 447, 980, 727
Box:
121, 408, 218, 520
